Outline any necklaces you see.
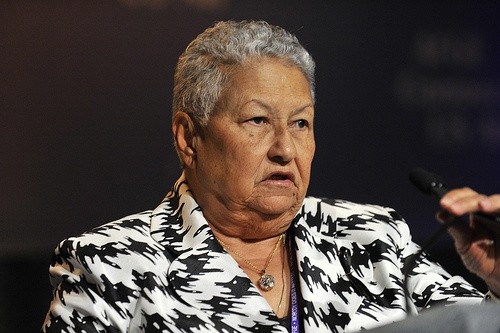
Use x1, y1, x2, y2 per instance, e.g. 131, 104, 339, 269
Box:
213, 233, 284, 291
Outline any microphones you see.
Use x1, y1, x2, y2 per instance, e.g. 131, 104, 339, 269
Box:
410, 167, 499, 240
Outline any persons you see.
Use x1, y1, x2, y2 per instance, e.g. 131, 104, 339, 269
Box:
39, 21, 500, 333
